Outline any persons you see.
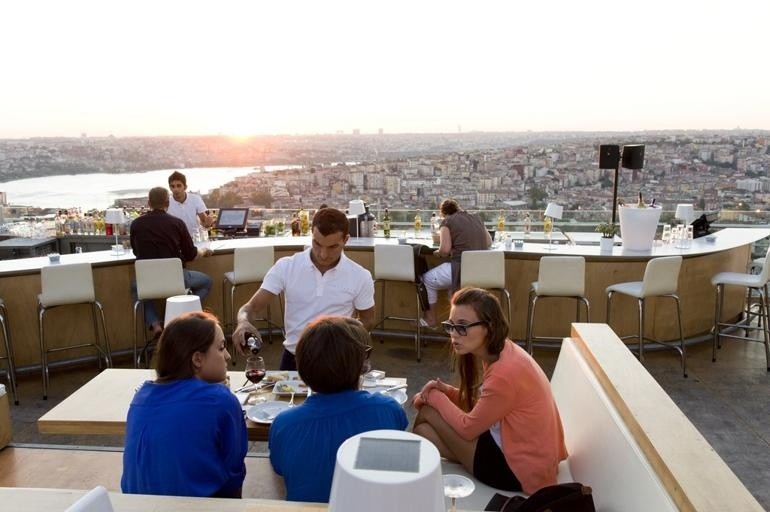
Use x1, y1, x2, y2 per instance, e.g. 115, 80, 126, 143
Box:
120, 311, 249, 499
267, 314, 410, 504
129, 187, 212, 340
411, 198, 493, 330
166, 170, 217, 247
412, 286, 570, 495
232, 207, 377, 374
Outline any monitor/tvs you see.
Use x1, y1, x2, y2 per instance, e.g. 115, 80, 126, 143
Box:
216, 208, 249, 228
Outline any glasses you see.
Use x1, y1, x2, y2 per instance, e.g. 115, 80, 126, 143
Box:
363, 344, 374, 361
441, 321, 484, 336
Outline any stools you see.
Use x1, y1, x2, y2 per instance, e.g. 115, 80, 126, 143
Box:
0, 379, 13, 454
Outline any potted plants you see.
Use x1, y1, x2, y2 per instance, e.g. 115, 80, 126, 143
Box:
591, 222, 619, 253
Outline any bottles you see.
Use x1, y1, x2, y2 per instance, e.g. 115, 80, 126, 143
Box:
415, 208, 421, 235
383, 207, 391, 238
505, 234, 512, 250
524, 213, 531, 235
240, 332, 261, 357
290, 208, 311, 236
55, 206, 147, 236
498, 209, 505, 232
544, 215, 552, 236
431, 212, 438, 233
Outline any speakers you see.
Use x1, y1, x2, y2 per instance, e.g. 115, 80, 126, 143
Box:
622, 144, 645, 169
600, 145, 619, 168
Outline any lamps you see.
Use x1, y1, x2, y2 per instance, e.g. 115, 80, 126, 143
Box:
671, 202, 696, 251
348, 198, 367, 238
328, 429, 447, 512
103, 207, 128, 258
543, 201, 565, 251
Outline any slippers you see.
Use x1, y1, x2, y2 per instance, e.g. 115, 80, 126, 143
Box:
419, 318, 439, 331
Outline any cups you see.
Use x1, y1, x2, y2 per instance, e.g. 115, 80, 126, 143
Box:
663, 222, 695, 249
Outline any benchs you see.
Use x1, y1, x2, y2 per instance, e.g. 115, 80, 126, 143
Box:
1, 485, 333, 512
437, 321, 769, 512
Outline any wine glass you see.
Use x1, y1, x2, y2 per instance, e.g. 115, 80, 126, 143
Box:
244, 357, 267, 405
442, 473, 476, 510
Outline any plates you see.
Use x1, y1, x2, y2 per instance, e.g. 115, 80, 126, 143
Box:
271, 380, 317, 396
246, 401, 296, 425
365, 385, 408, 406
